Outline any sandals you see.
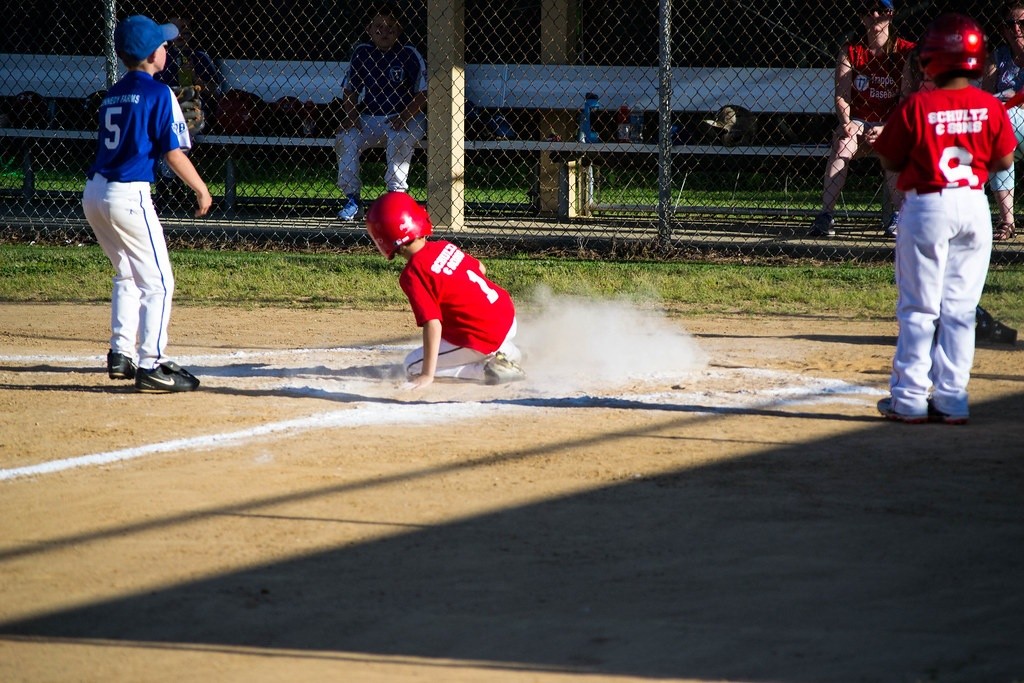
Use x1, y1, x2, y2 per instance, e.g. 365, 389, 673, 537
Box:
994, 222, 1015, 240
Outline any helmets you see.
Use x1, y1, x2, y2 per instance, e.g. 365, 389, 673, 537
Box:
918, 14, 986, 79
366, 191, 431, 260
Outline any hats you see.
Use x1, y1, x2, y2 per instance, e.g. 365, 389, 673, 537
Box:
114, 15, 179, 61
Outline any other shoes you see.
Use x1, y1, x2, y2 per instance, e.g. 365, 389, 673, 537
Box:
156, 192, 170, 213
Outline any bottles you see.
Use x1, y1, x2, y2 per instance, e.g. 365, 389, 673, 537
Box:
630, 103, 644, 143
616, 106, 632, 143
582, 92, 602, 142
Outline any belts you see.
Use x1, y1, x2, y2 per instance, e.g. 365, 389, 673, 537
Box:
917, 185, 982, 195
88, 172, 130, 183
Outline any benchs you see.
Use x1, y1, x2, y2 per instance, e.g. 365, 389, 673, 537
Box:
0, 53, 1007, 210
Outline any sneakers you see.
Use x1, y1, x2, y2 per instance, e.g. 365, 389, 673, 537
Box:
877, 398, 928, 423
883, 211, 899, 237
486, 350, 526, 383
135, 361, 200, 394
811, 213, 836, 235
108, 349, 135, 380
337, 198, 363, 220
928, 398, 969, 425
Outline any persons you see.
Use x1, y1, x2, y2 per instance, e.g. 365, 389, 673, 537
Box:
335, 9, 428, 221
365, 191, 528, 392
79, 15, 225, 394
984, 0, 1024, 240
805, 0, 919, 238
868, 17, 1016, 424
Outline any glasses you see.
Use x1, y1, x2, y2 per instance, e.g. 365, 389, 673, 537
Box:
1002, 19, 1024, 29
863, 8, 890, 16
